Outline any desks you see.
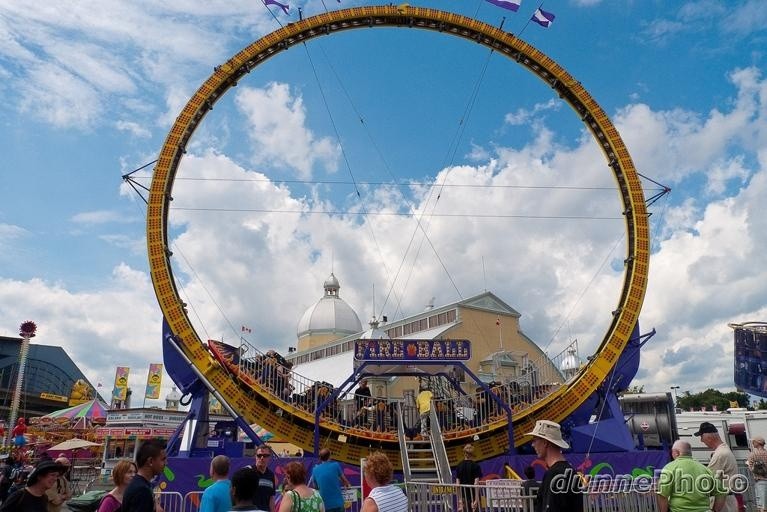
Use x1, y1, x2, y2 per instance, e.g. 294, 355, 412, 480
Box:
72, 465, 92, 494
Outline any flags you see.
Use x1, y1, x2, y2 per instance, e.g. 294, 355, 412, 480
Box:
261, 0, 291, 18
481, 0, 522, 13
241, 326, 251, 333
529, 8, 556, 30
144, 363, 163, 399
112, 366, 129, 400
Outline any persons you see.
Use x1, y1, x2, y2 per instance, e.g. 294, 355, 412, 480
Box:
0, 451, 73, 511
748, 436, 767, 512
655, 439, 725, 512
12, 417, 28, 448
121, 439, 167, 512
241, 445, 276, 512
359, 451, 413, 512
284, 450, 290, 458
279, 461, 325, 512
694, 423, 745, 512
238, 349, 545, 438
296, 449, 302, 457
278, 449, 285, 458
95, 459, 137, 512
198, 454, 233, 511
275, 477, 293, 512
521, 466, 540, 512
311, 448, 351, 512
455, 443, 483, 512
523, 419, 584, 511
226, 468, 269, 512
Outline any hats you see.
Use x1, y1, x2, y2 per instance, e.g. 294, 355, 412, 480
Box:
749, 436, 765, 445
27, 461, 63, 486
524, 418, 570, 450
694, 423, 718, 435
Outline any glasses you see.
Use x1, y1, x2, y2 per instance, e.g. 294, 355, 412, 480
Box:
257, 454, 270, 457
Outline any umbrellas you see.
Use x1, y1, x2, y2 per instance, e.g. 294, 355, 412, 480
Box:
47, 437, 103, 480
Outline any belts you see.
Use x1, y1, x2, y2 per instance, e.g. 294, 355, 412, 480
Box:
710, 490, 734, 497
